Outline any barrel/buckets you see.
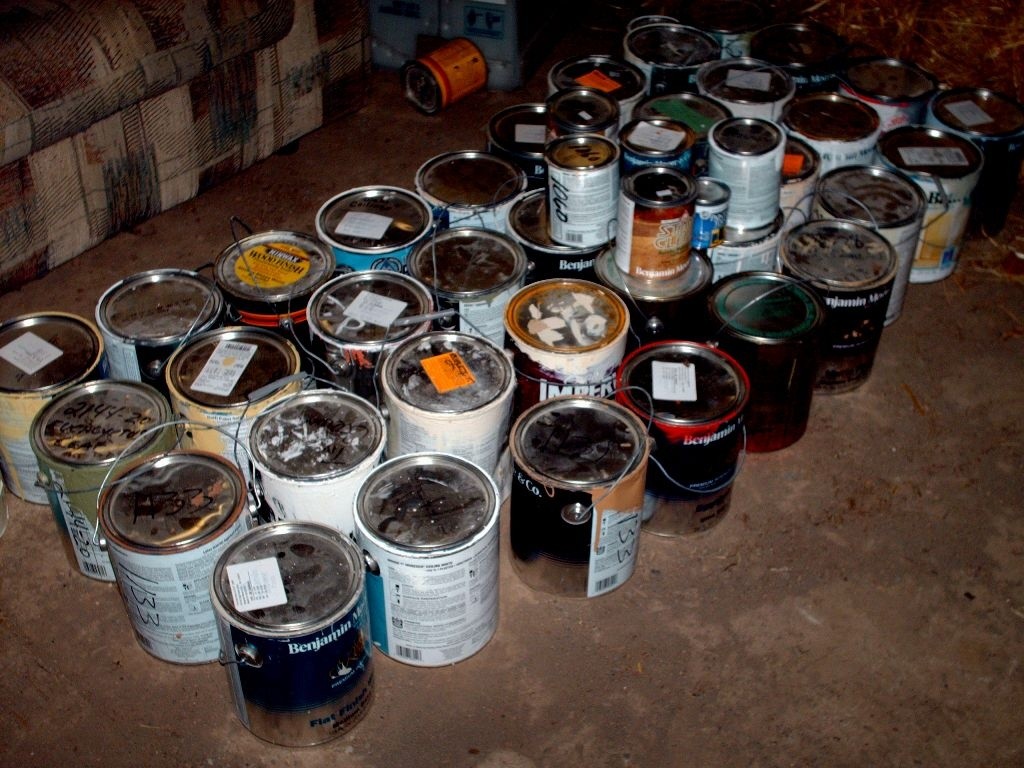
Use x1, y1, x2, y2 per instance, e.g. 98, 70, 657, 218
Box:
1, 1, 1024, 747
